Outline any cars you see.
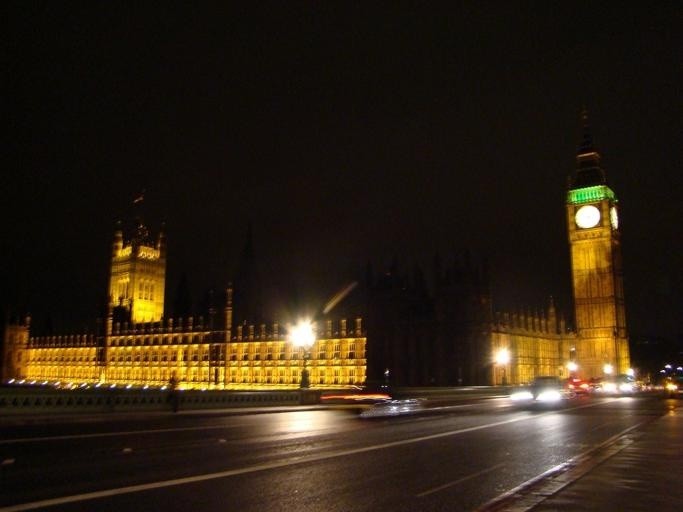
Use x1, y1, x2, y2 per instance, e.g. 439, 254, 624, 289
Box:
506, 373, 680, 406
359, 398, 417, 418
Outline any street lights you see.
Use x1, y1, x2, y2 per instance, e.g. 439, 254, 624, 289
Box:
494, 347, 511, 386
289, 321, 319, 389
